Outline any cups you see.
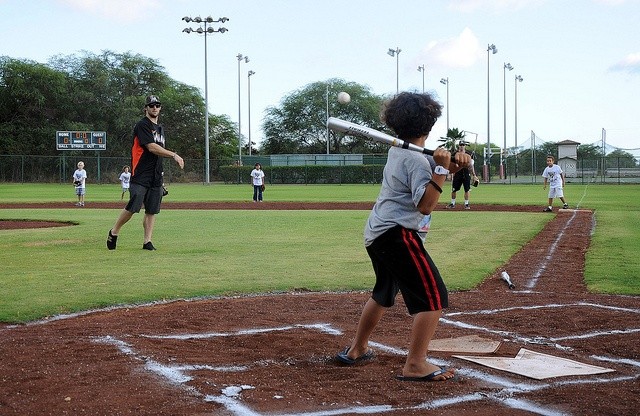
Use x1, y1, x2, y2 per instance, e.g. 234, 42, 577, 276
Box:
149, 104, 161, 107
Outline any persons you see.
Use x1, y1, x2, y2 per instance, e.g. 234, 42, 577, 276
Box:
106, 94, 185, 251
542, 156, 568, 212
72, 161, 87, 206
250, 163, 265, 203
337, 89, 471, 383
119, 165, 132, 199
446, 141, 480, 209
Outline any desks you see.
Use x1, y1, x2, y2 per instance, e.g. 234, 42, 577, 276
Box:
387, 46, 402, 95
440, 77, 449, 138
503, 62, 514, 179
417, 64, 424, 94
248, 70, 255, 155
514, 75, 524, 178
182, 15, 229, 185
236, 53, 250, 184
486, 43, 498, 182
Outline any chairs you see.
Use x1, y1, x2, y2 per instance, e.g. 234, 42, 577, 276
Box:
543, 207, 552, 212
107, 228, 118, 249
143, 242, 156, 250
446, 203, 455, 208
563, 203, 568, 208
464, 203, 470, 210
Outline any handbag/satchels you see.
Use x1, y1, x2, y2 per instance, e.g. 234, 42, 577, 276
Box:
428, 179, 443, 193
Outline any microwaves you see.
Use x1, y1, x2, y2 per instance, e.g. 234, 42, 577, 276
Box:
326, 117, 475, 170
559, 209, 592, 213
501, 272, 515, 290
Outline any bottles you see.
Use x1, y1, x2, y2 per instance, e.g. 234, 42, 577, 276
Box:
144, 94, 161, 105
459, 140, 465, 145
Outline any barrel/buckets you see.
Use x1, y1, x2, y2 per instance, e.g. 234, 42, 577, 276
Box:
337, 344, 374, 361
396, 361, 458, 381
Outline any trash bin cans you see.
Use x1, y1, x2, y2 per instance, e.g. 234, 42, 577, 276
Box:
434, 165, 449, 175
